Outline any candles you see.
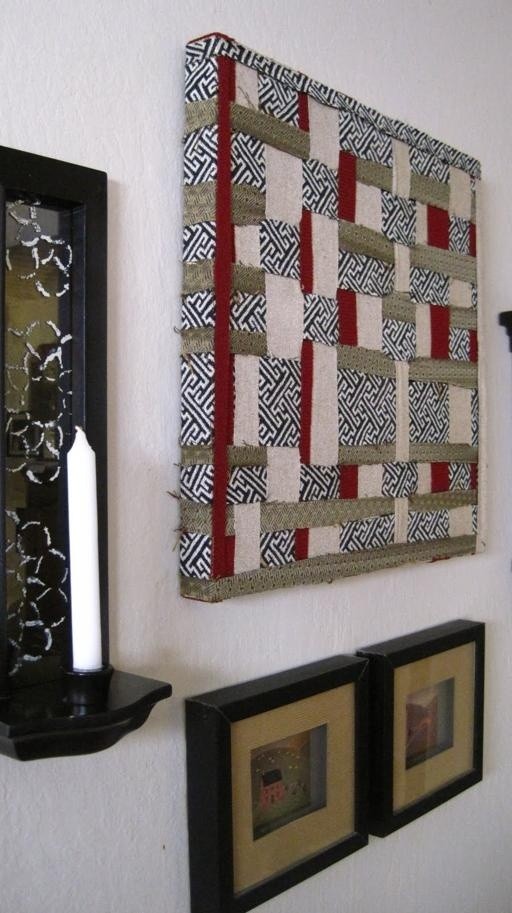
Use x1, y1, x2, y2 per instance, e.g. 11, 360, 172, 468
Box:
65, 424, 106, 675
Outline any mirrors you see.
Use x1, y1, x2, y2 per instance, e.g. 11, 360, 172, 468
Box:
0, 146, 110, 689
